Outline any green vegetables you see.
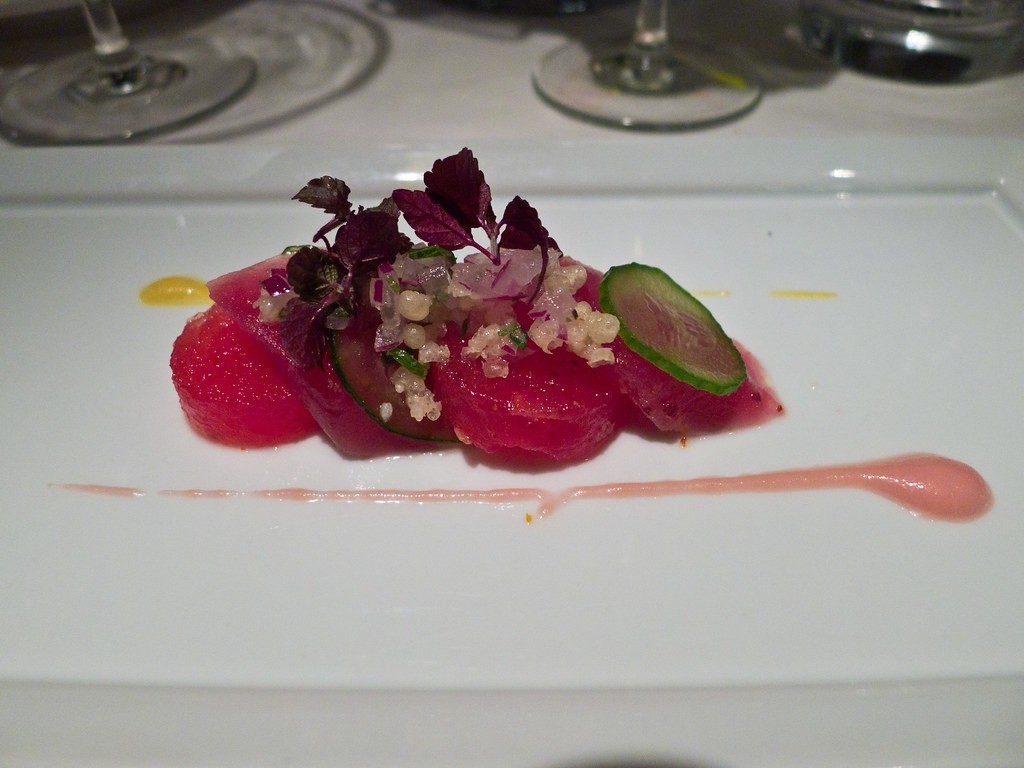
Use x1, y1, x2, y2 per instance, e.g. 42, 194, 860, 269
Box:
281, 148, 561, 369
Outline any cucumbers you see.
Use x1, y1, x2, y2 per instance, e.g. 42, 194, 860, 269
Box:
597, 261, 748, 395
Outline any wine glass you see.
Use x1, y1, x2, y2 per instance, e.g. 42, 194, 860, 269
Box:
526, 0, 763, 130
0, 0, 260, 147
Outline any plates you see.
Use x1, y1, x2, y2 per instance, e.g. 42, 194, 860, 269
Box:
0, 136, 1024, 766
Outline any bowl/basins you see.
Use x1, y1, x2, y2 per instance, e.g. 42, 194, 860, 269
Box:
804, 1, 1024, 84
